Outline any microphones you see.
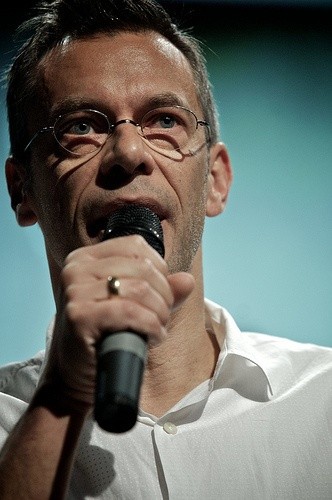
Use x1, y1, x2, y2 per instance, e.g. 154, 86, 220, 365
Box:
93, 206, 167, 434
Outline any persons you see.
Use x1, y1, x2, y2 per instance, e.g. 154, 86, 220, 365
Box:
0, 0, 332, 500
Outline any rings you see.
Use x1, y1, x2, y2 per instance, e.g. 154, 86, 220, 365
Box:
107, 274, 120, 298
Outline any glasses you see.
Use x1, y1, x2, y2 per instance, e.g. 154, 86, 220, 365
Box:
22, 105, 214, 155
